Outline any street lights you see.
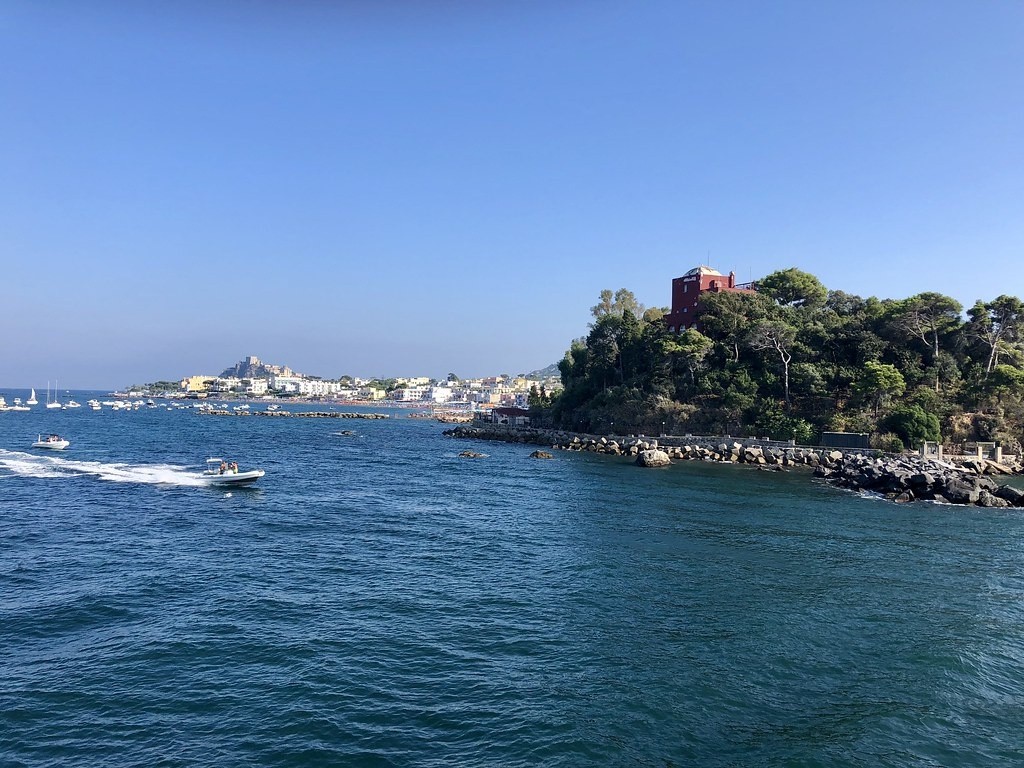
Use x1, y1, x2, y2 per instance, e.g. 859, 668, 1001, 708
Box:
961, 438, 966, 454
611, 422, 613, 434
662, 421, 665, 437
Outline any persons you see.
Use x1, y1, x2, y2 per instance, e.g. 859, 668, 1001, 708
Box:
220, 461, 226, 475
46, 435, 64, 443
231, 462, 238, 474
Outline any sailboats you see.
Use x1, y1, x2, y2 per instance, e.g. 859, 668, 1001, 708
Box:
26, 388, 38, 405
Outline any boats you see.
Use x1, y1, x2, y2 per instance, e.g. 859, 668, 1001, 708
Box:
194, 456, 265, 488
0, 397, 30, 413
233, 404, 250, 411
266, 403, 281, 411
45, 380, 82, 410
87, 399, 145, 411
147, 399, 228, 411
32, 434, 69, 450
